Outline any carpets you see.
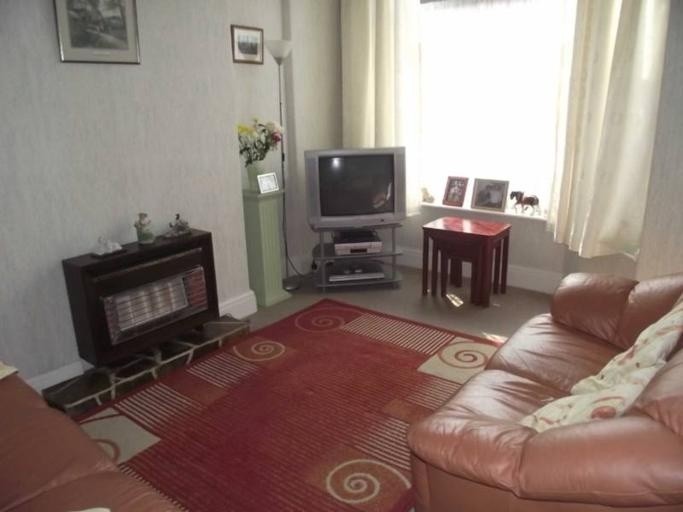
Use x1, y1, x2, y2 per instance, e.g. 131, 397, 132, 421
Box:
71, 297, 507, 511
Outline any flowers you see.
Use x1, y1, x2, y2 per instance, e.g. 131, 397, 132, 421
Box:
234, 115, 286, 166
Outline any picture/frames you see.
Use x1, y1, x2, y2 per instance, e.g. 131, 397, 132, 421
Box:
255, 172, 279, 194
230, 24, 263, 65
52, 0, 140, 64
441, 175, 510, 212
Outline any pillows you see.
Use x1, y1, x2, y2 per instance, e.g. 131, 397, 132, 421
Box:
516, 304, 682, 432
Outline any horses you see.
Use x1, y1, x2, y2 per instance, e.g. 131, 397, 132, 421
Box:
510, 191, 541, 216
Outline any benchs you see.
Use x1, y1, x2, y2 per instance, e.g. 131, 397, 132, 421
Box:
0, 360, 186, 512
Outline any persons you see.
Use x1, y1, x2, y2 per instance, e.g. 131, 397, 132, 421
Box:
489, 184, 499, 207
476, 185, 491, 206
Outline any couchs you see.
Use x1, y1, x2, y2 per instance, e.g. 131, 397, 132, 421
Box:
404, 270, 683, 512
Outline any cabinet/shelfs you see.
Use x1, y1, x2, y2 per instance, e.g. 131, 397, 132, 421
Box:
309, 222, 404, 292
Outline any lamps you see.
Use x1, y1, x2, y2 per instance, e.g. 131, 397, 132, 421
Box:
265, 38, 302, 293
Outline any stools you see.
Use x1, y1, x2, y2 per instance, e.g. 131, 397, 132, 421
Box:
418, 216, 512, 310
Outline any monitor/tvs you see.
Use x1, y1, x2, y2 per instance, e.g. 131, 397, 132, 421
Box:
304, 146, 406, 229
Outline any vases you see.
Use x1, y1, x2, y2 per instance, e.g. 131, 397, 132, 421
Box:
245, 158, 262, 193
